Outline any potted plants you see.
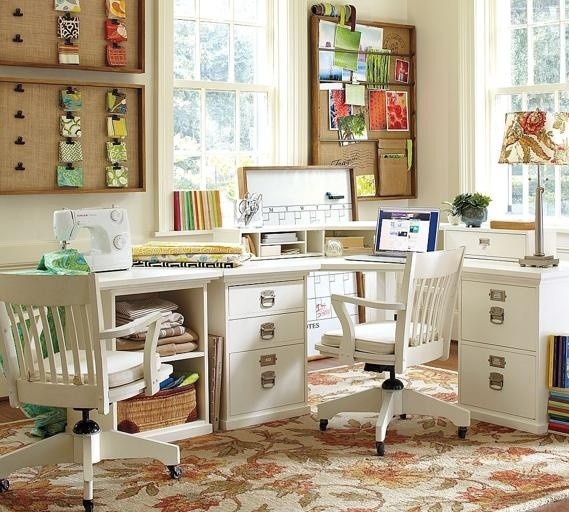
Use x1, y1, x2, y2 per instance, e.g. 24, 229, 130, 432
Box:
441, 192, 493, 227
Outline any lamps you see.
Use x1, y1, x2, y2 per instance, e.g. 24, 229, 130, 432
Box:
498, 109, 569, 268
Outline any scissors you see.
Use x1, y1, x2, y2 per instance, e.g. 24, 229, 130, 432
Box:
238, 192, 263, 227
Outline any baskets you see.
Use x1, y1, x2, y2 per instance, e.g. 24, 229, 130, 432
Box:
117, 383, 197, 429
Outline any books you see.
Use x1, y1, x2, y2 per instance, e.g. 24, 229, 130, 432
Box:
262, 233, 299, 244
173, 189, 224, 230
547, 333, 569, 437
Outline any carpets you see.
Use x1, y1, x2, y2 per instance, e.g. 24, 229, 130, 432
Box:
0, 361, 569, 511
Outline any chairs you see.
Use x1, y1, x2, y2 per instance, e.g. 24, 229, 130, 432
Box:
0, 274, 182, 511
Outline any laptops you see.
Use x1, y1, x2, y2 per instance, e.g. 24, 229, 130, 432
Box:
345, 207, 441, 264
